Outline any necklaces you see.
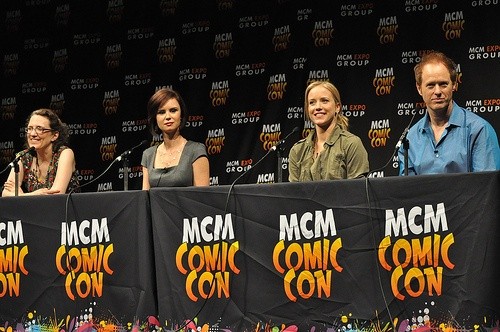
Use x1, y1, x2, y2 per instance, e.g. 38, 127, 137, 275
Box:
158, 139, 187, 165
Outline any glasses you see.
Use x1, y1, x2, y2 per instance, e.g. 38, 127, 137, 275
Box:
24, 126, 55, 134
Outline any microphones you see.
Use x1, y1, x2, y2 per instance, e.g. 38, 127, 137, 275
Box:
268, 126, 299, 152
13, 146, 35, 161
394, 105, 420, 151
118, 139, 148, 160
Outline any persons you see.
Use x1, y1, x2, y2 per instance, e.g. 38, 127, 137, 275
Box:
141, 89, 211, 190
288, 80, 370, 182
1, 109, 81, 197
398, 53, 500, 176
